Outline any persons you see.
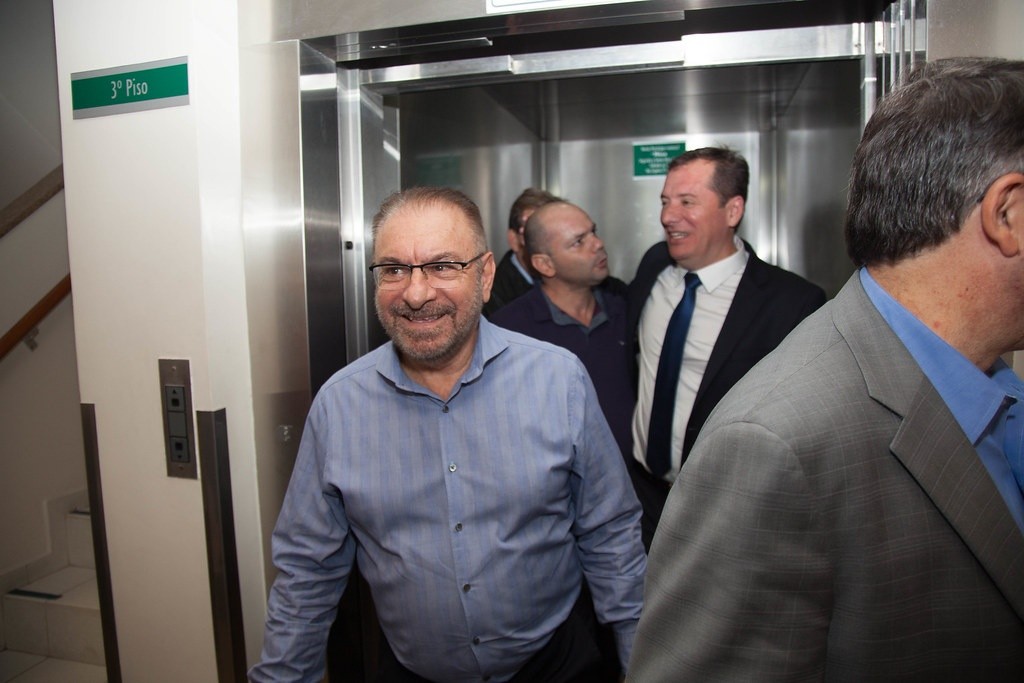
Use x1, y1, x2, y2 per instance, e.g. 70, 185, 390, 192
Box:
483, 147, 827, 553
624, 54, 1024, 683
247, 186, 647, 683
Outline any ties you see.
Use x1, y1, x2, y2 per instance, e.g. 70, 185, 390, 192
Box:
645, 273, 701, 478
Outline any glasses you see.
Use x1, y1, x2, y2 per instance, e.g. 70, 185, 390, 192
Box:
367, 251, 488, 291
